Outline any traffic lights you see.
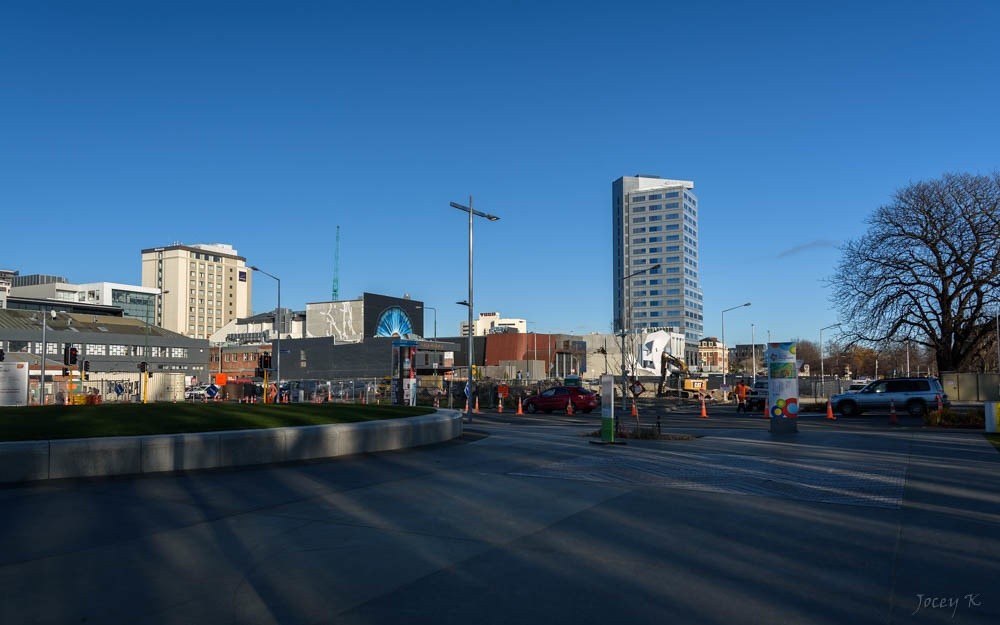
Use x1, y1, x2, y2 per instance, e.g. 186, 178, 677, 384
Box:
262, 355, 268, 367
71, 348, 78, 364
141, 362, 146, 372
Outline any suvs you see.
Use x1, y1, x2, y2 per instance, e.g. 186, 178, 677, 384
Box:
745, 380, 769, 408
831, 376, 948, 418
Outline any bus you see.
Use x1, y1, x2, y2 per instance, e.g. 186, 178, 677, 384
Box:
280, 377, 390, 400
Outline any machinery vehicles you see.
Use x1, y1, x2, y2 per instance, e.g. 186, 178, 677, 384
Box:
657, 351, 711, 399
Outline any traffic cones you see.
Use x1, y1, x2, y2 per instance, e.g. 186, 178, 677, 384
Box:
566, 397, 573, 415
172, 392, 365, 406
825, 398, 836, 419
698, 397, 709, 417
516, 397, 525, 415
889, 400, 897, 423
630, 400, 638, 416
937, 398, 943, 413
765, 398, 769, 416
433, 394, 438, 408
465, 399, 468, 412
497, 398, 504, 413
474, 395, 481, 411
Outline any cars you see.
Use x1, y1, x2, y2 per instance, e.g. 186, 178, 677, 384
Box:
710, 371, 752, 379
185, 385, 219, 398
523, 385, 597, 415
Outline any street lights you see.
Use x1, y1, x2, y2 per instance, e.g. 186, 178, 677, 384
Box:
449, 196, 499, 422
620, 263, 662, 412
145, 290, 169, 359
722, 302, 751, 384
252, 266, 281, 403
820, 323, 842, 398
415, 306, 438, 375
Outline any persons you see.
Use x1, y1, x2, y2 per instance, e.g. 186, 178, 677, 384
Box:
734, 379, 750, 418
26, 354, 289, 406
516, 369, 522, 380
